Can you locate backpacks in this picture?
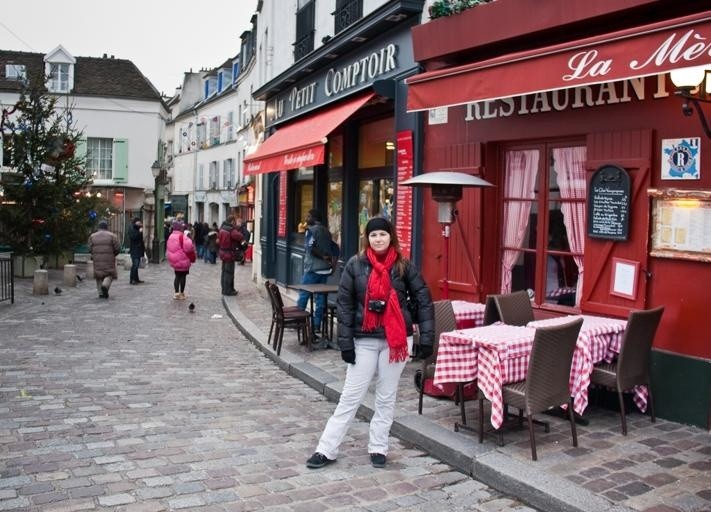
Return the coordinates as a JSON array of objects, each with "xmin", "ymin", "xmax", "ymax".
[
  {"xmin": 185, "ymin": 249, "xmax": 197, "ymax": 263},
  {"xmin": 235, "ymin": 241, "xmax": 248, "ymax": 263}
]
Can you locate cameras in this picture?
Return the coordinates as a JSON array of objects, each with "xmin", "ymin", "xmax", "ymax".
[{"xmin": 368, "ymin": 299, "xmax": 386, "ymax": 316}]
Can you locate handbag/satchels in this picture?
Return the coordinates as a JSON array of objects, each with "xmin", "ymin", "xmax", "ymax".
[
  {"xmin": 407, "ymin": 298, "xmax": 420, "ymax": 324},
  {"xmin": 139, "ymin": 257, "xmax": 146, "ymax": 269}
]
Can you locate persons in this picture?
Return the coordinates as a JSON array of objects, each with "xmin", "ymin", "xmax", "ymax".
[
  {"xmin": 165, "ymin": 215, "xmax": 249, "ymax": 300},
  {"xmin": 306, "ymin": 218, "xmax": 435, "ymax": 469},
  {"xmin": 128, "ymin": 218, "xmax": 144, "ymax": 282},
  {"xmin": 297, "ymin": 210, "xmax": 339, "ymax": 333},
  {"xmin": 88, "ymin": 221, "xmax": 121, "ymax": 298}
]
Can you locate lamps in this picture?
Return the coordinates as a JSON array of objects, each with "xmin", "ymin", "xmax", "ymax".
[
  {"xmin": 670, "ymin": 65, "xmax": 711, "ymax": 117},
  {"xmin": 398, "ymin": 172, "xmax": 496, "ymax": 398}
]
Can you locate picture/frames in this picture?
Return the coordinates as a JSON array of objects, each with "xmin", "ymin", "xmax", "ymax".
[
  {"xmin": 609, "ymin": 257, "xmax": 640, "ymax": 300},
  {"xmin": 647, "ymin": 189, "xmax": 711, "ymax": 262}
]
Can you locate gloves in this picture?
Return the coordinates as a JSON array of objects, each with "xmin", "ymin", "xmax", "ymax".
[
  {"xmin": 416, "ymin": 346, "xmax": 434, "ymax": 359},
  {"xmin": 341, "ymin": 349, "xmax": 356, "ymax": 365}
]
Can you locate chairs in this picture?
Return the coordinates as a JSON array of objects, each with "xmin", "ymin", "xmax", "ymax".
[
  {"xmin": 418, "ymin": 300, "xmax": 467, "ymax": 423},
  {"xmin": 479, "ymin": 318, "xmax": 584, "ymax": 460},
  {"xmin": 589, "ymin": 306, "xmax": 665, "ymax": 435},
  {"xmin": 483, "ymin": 294, "xmax": 500, "ymax": 326},
  {"xmin": 330, "ymin": 313, "xmax": 339, "ymax": 345},
  {"xmin": 268, "ymin": 284, "xmax": 313, "ymax": 355},
  {"xmin": 266, "ymin": 281, "xmax": 304, "ymax": 344},
  {"xmin": 494, "ymin": 290, "xmax": 535, "ymax": 327},
  {"xmin": 322, "ymin": 303, "xmax": 338, "ymax": 338}
]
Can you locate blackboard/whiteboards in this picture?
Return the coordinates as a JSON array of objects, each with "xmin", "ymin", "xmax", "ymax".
[{"xmin": 587, "ymin": 164, "xmax": 632, "ymax": 243}]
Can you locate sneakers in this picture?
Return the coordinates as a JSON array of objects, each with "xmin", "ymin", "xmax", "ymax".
[
  {"xmin": 99, "ymin": 295, "xmax": 103, "ymax": 299},
  {"xmin": 370, "ymin": 453, "xmax": 386, "ymax": 468},
  {"xmin": 306, "ymin": 453, "xmax": 337, "ymax": 469},
  {"xmin": 179, "ymin": 293, "xmax": 186, "ymax": 300},
  {"xmin": 101, "ymin": 285, "xmax": 109, "ymax": 298},
  {"xmin": 173, "ymin": 293, "xmax": 179, "ymax": 300}
]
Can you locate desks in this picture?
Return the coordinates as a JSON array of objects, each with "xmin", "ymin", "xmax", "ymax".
[{"xmin": 285, "ymin": 283, "xmax": 339, "ymax": 348}]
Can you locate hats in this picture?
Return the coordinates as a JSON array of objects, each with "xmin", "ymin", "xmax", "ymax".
[
  {"xmin": 98, "ymin": 221, "xmax": 107, "ymax": 229},
  {"xmin": 172, "ymin": 220, "xmax": 182, "ymax": 230}
]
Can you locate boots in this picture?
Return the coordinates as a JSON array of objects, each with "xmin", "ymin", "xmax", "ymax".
[{"xmin": 129, "ymin": 276, "xmax": 144, "ymax": 285}]
